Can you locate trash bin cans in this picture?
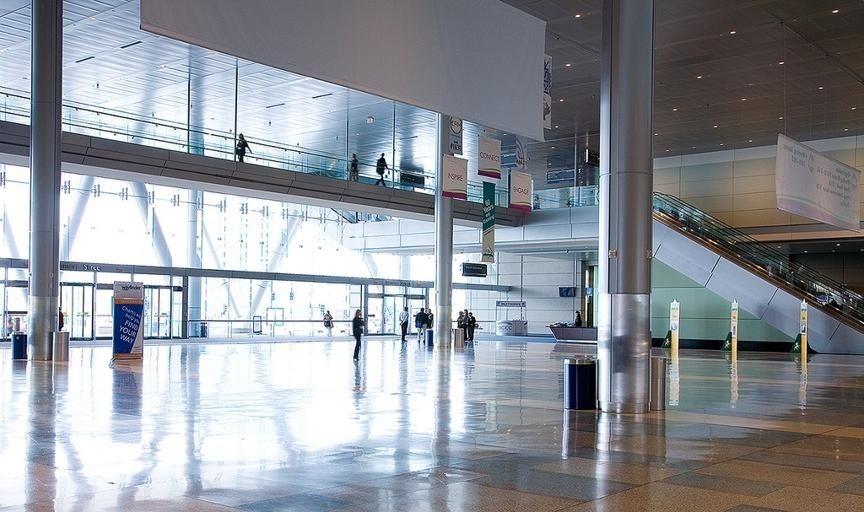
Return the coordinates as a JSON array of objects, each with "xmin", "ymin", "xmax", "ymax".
[
  {"xmin": 452, "ymin": 328, "xmax": 464, "ymax": 350},
  {"xmin": 425, "ymin": 329, "xmax": 433, "ymax": 347},
  {"xmin": 52, "ymin": 331, "xmax": 68, "ymax": 362},
  {"xmin": 563, "ymin": 358, "xmax": 597, "ymax": 411},
  {"xmin": 649, "ymin": 357, "xmax": 666, "ymax": 411},
  {"xmin": 201, "ymin": 323, "xmax": 207, "ymax": 337},
  {"xmin": 10, "ymin": 331, "xmax": 28, "ymax": 359}
]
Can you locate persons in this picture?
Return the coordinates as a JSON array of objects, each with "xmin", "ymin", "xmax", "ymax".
[
  {"xmin": 350, "ymin": 152, "xmax": 360, "ymax": 182},
  {"xmin": 457, "ymin": 311, "xmax": 465, "ymax": 328},
  {"xmin": 236, "ymin": 132, "xmax": 253, "ymax": 163},
  {"xmin": 459, "ymin": 308, "xmax": 471, "ymax": 343},
  {"xmin": 351, "ymin": 308, "xmax": 365, "ymax": 361},
  {"xmin": 574, "ymin": 310, "xmax": 582, "ymax": 328},
  {"xmin": 322, "ymin": 310, "xmax": 335, "ymax": 337},
  {"xmin": 373, "ymin": 152, "xmax": 390, "ymax": 188},
  {"xmin": 426, "ymin": 309, "xmax": 433, "ymax": 329},
  {"xmin": 399, "ymin": 306, "xmax": 409, "ymax": 342},
  {"xmin": 468, "ymin": 311, "xmax": 476, "ymax": 341},
  {"xmin": 414, "ymin": 307, "xmax": 430, "ymax": 344}
]
